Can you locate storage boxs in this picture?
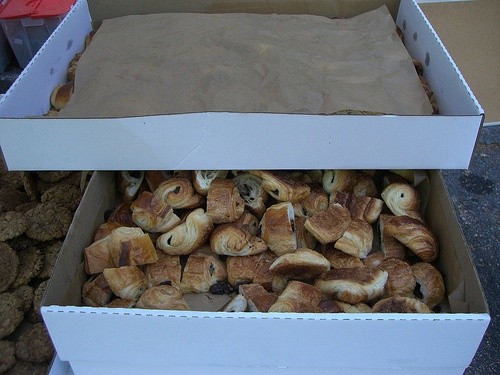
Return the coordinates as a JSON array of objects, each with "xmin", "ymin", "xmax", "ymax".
[
  {"xmin": 1, "ymin": 1, "xmax": 81, "ymax": 75},
  {"xmin": 0, "ymin": 0, "xmax": 491, "ymax": 374}
]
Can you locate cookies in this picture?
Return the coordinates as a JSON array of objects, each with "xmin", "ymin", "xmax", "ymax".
[{"xmin": 0, "ymin": 146, "xmax": 95, "ymax": 375}]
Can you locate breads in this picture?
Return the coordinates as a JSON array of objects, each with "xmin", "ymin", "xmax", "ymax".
[
  {"xmin": 412, "ymin": 59, "xmax": 439, "ymax": 115},
  {"xmin": 81, "ymin": 170, "xmax": 445, "ymax": 313},
  {"xmin": 42, "ymin": 31, "xmax": 97, "ymax": 118},
  {"xmin": 396, "ymin": 25, "xmax": 404, "ymax": 44}
]
